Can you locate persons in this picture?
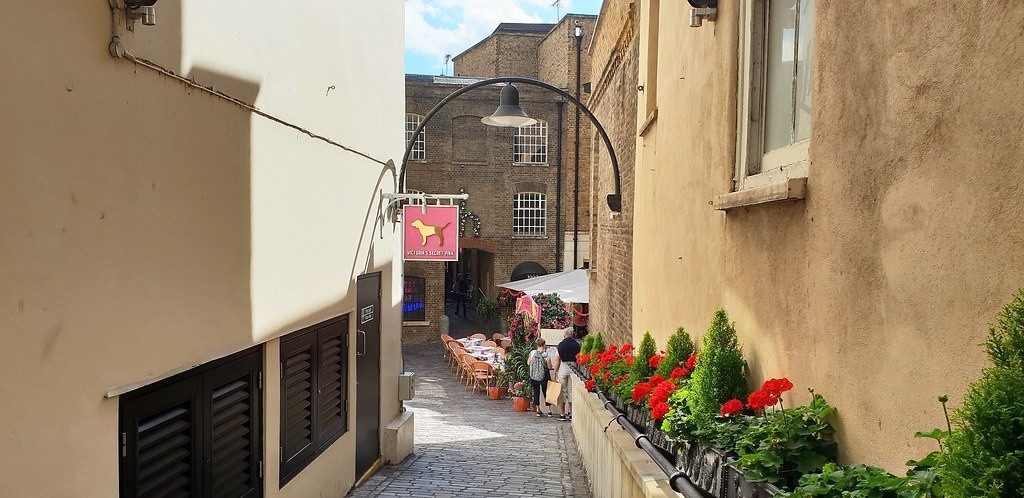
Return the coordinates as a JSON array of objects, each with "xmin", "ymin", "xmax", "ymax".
[
  {"xmin": 529, "ymin": 303, "xmax": 589, "ymax": 421},
  {"xmin": 453, "ymin": 273, "xmax": 468, "ymax": 319}
]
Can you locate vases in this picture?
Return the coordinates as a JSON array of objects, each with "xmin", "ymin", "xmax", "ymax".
[
  {"xmin": 513, "ymin": 397, "xmax": 530, "ymax": 411},
  {"xmin": 489, "ymin": 387, "xmax": 503, "ymax": 399},
  {"xmin": 541, "ymin": 327, "xmax": 573, "ymax": 346},
  {"xmin": 577, "ymin": 364, "xmax": 802, "ymax": 498}
]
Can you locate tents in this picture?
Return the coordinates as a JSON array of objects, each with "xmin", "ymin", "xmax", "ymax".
[{"xmin": 496, "ymin": 269, "xmax": 590, "ymax": 303}]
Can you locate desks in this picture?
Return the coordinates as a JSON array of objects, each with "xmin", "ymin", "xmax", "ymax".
[{"xmin": 457, "ymin": 337, "xmax": 511, "ymax": 372}]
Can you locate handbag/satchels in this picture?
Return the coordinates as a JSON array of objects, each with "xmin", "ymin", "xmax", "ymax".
[
  {"xmin": 544, "ymin": 366, "xmax": 550, "ymax": 379},
  {"xmin": 546, "ymin": 380, "xmax": 564, "ymax": 407}
]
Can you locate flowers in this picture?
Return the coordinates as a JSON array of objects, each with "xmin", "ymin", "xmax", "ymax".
[
  {"xmin": 576, "ymin": 307, "xmax": 837, "ymax": 490},
  {"xmin": 532, "ymin": 292, "xmax": 573, "ymax": 330},
  {"xmin": 488, "ymin": 310, "xmax": 541, "ymax": 402}
]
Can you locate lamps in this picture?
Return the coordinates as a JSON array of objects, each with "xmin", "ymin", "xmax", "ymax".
[{"xmin": 481, "ymin": 81, "xmax": 537, "ymax": 127}]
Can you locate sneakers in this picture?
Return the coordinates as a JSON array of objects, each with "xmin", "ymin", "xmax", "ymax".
[
  {"xmin": 555, "ymin": 413, "xmax": 566, "ymax": 421},
  {"xmin": 547, "ymin": 412, "xmax": 552, "ymax": 417},
  {"xmin": 570, "ymin": 416, "xmax": 572, "ymax": 420},
  {"xmin": 537, "ymin": 411, "xmax": 544, "ymax": 417}
]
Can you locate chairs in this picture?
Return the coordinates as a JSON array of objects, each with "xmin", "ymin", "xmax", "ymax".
[
  {"xmin": 471, "ymin": 333, "xmax": 512, "ymax": 360},
  {"xmin": 441, "ymin": 334, "xmax": 495, "ymax": 396}
]
